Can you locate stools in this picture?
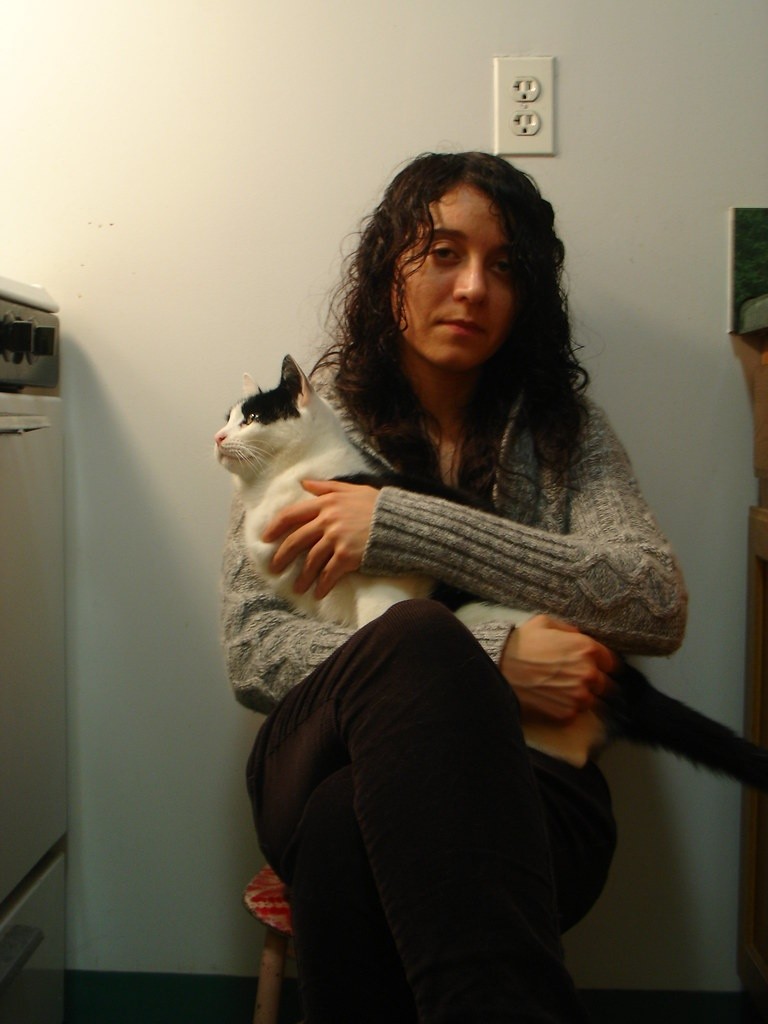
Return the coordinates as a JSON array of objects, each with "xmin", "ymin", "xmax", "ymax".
[{"xmin": 241, "ymin": 862, "xmax": 293, "ymax": 1024}]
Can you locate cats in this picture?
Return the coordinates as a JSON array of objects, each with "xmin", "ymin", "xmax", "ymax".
[{"xmin": 213, "ymin": 348, "xmax": 768, "ymax": 794}]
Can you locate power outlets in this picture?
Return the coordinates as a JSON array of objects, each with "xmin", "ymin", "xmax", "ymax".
[{"xmin": 492, "ymin": 55, "xmax": 558, "ymax": 156}]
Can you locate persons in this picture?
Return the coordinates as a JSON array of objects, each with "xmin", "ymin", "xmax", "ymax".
[{"xmin": 219, "ymin": 151, "xmax": 688, "ymax": 1024}]
